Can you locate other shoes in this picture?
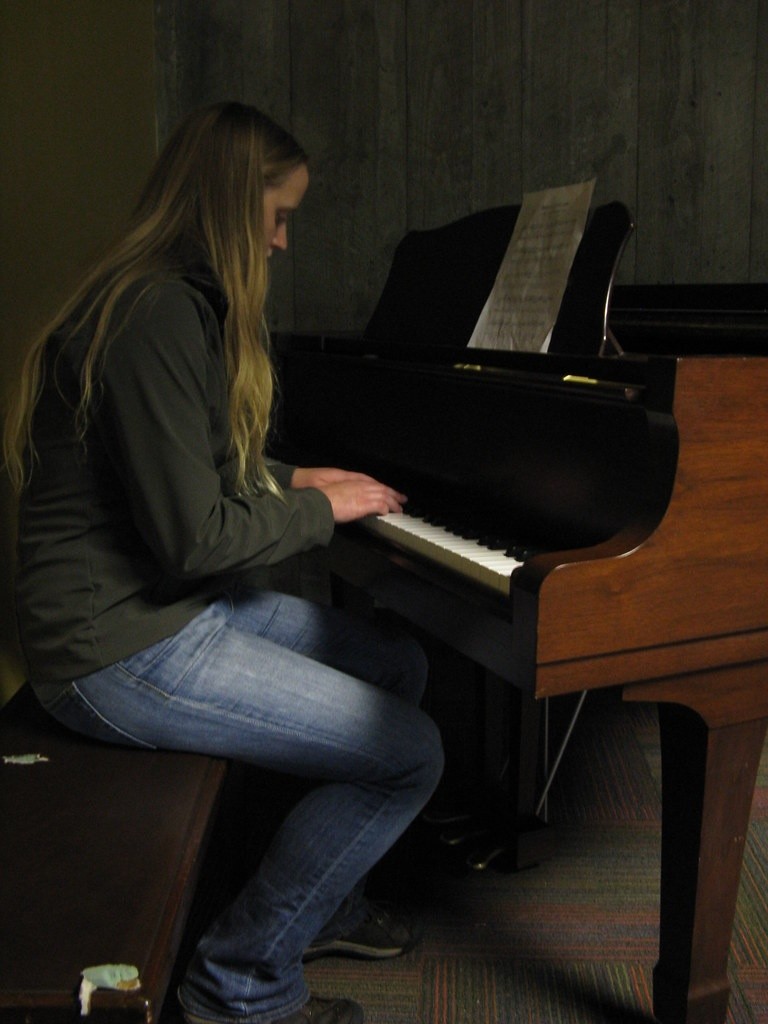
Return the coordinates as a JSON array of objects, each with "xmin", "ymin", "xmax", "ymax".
[
  {"xmin": 302, "ymin": 915, "xmax": 404, "ymax": 958},
  {"xmin": 268, "ymin": 993, "xmax": 367, "ymax": 1024}
]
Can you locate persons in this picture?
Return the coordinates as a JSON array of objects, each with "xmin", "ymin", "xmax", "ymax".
[{"xmin": 1, "ymin": 102, "xmax": 452, "ymax": 1024}]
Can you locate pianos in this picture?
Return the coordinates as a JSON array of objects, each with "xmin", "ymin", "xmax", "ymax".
[{"xmin": 267, "ymin": 278, "xmax": 768, "ymax": 1024}]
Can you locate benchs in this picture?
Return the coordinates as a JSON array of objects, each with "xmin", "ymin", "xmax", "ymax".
[{"xmin": 1, "ymin": 676, "xmax": 238, "ymax": 1024}]
